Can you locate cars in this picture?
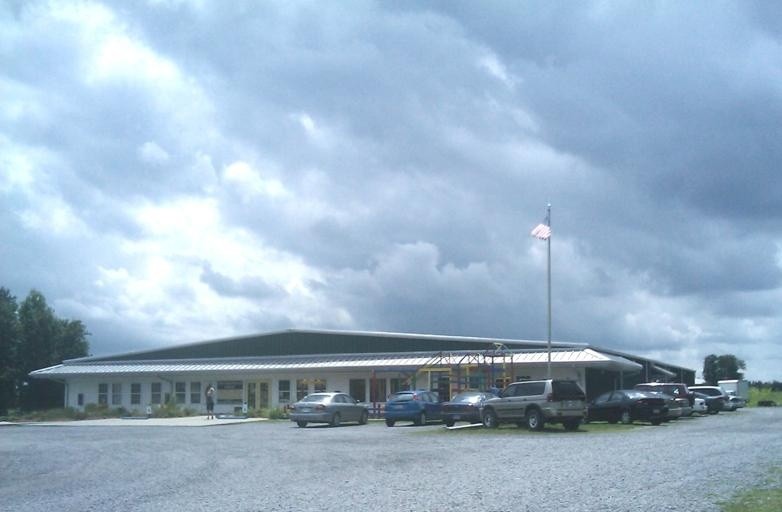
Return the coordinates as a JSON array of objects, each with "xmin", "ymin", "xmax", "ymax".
[
  {"xmin": 439, "ymin": 392, "xmax": 496, "ymax": 427},
  {"xmin": 689, "ymin": 380, "xmax": 747, "ymax": 415},
  {"xmin": 583, "ymin": 390, "xmax": 669, "ymax": 425},
  {"xmin": 648, "ymin": 390, "xmax": 691, "ymax": 420},
  {"xmin": 289, "ymin": 392, "xmax": 369, "ymax": 427},
  {"xmin": 383, "ymin": 390, "xmax": 446, "ymax": 426}
]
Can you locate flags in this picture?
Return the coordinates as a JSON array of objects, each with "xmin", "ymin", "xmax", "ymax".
[{"xmin": 530, "ymin": 211, "xmax": 552, "ymax": 241}]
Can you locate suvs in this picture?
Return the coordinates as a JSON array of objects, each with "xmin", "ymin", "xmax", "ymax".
[
  {"xmin": 632, "ymin": 382, "xmax": 696, "ymax": 416},
  {"xmin": 479, "ymin": 379, "xmax": 588, "ymax": 431}
]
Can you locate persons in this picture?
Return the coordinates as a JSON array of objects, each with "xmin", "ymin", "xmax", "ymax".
[{"xmin": 206, "ymin": 387, "xmax": 215, "ymax": 420}]
